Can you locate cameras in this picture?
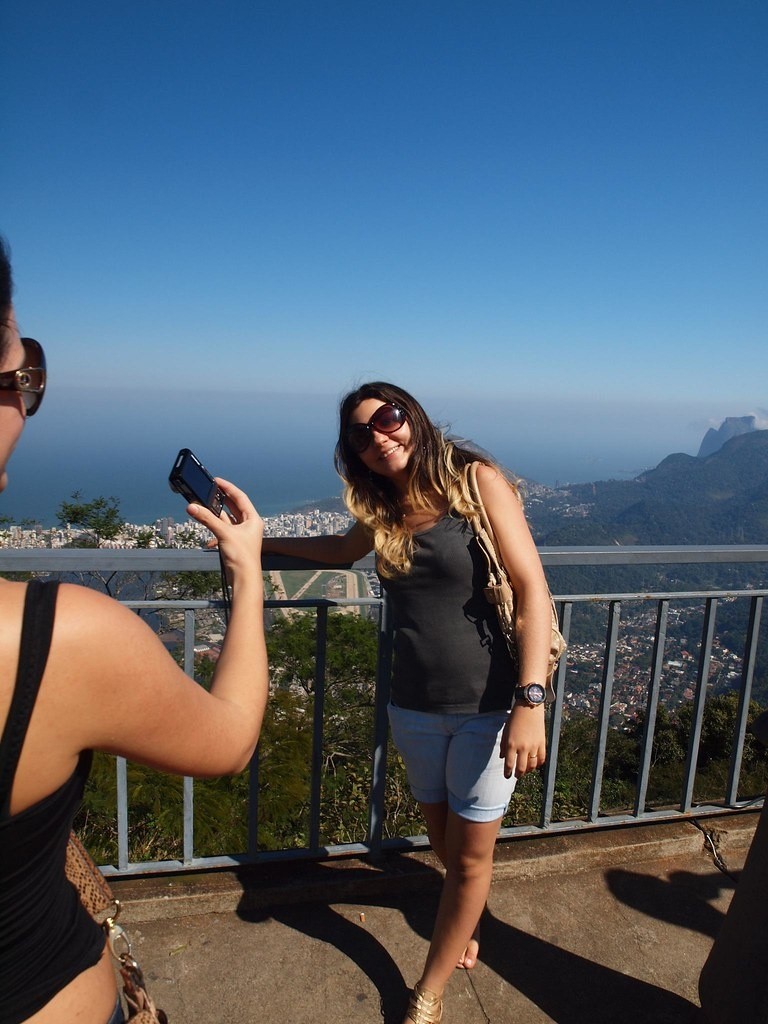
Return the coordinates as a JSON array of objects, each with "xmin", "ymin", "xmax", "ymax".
[{"xmin": 168, "ymin": 448, "xmax": 228, "ymax": 518}]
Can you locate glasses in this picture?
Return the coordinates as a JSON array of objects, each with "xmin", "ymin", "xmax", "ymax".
[
  {"xmin": 0, "ymin": 338, "xmax": 47, "ymax": 416},
  {"xmin": 347, "ymin": 403, "xmax": 406, "ymax": 452}
]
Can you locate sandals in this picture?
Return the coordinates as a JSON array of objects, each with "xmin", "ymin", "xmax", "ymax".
[{"xmin": 407, "ymin": 981, "xmax": 443, "ymax": 1024}]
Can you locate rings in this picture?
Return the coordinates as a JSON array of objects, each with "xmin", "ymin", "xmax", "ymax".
[{"xmin": 529, "ymin": 755, "xmax": 537, "ymax": 758}]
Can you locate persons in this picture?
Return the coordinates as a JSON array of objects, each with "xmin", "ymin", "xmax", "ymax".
[
  {"xmin": 204, "ymin": 381, "xmax": 554, "ymax": 1024},
  {"xmin": 1, "ymin": 240, "xmax": 269, "ymax": 1023}
]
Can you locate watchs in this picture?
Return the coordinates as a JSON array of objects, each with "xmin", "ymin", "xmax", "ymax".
[{"xmin": 515, "ymin": 682, "xmax": 547, "ymax": 708}]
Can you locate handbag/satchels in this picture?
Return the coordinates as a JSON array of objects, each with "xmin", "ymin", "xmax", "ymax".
[
  {"xmin": 459, "ymin": 460, "xmax": 567, "ymax": 675},
  {"xmin": 119, "ymin": 970, "xmax": 160, "ymax": 1024}
]
